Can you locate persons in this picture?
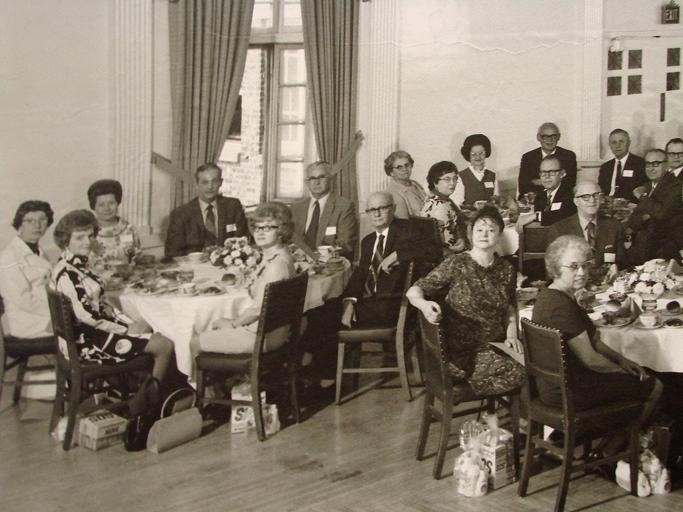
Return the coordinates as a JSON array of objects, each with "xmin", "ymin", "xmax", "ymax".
[
  {"xmin": 547, "ymin": 179, "xmax": 626, "ymax": 271},
  {"xmin": 628, "ymin": 148, "xmax": 683, "ymax": 271},
  {"xmin": 518, "ymin": 123, "xmax": 576, "ymax": 201},
  {"xmin": 534, "ymin": 154, "xmax": 577, "ymax": 225},
  {"xmin": 165, "ymin": 162, "xmax": 255, "ymax": 264},
  {"xmin": 47, "ymin": 206, "xmax": 173, "ymax": 417},
  {"xmin": 406, "ymin": 206, "xmax": 525, "ymax": 397},
  {"xmin": 284, "ymin": 160, "xmax": 359, "ymax": 266},
  {"xmin": 449, "ymin": 134, "xmax": 500, "ymax": 212},
  {"xmin": 87, "ymin": 179, "xmax": 154, "ymax": 283},
  {"xmin": 187, "ymin": 202, "xmax": 296, "ymax": 425},
  {"xmin": 385, "ymin": 151, "xmax": 428, "ymax": 218},
  {"xmin": 597, "ymin": 129, "xmax": 648, "ymax": 200},
  {"xmin": 665, "ymin": 138, "xmax": 683, "ymax": 180},
  {"xmin": 0, "ymin": 200, "xmax": 55, "ymax": 342},
  {"xmin": 421, "ymin": 161, "xmax": 470, "ymax": 255},
  {"xmin": 532, "ymin": 233, "xmax": 663, "ymax": 474},
  {"xmin": 302, "ymin": 192, "xmax": 431, "ymax": 404}
]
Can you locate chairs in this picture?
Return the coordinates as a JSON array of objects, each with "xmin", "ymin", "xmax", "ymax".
[
  {"xmin": 414, "ymin": 308, "xmax": 524, "ymax": 483},
  {"xmin": 195, "ymin": 269, "xmax": 308, "ymax": 442},
  {"xmin": 518, "ymin": 315, "xmax": 644, "ymax": 512},
  {"xmin": 335, "ymin": 258, "xmax": 425, "ymax": 405},
  {"xmin": 41, "ymin": 281, "xmax": 153, "ymax": 451},
  {"xmin": 0, "ymin": 295, "xmax": 57, "ymax": 404},
  {"xmin": 517, "ymin": 229, "xmax": 550, "ymax": 286}
]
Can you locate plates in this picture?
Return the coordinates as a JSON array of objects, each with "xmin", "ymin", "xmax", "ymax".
[
  {"xmin": 316, "ymin": 259, "xmax": 327, "ymax": 262},
  {"xmin": 129, "ymin": 251, "xmax": 242, "ymax": 299},
  {"xmin": 586, "ymin": 275, "xmax": 682, "ymax": 331}
]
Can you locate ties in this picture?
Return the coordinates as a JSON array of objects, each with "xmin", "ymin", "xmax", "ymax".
[
  {"xmin": 363, "ymin": 233, "xmax": 385, "ymax": 297},
  {"xmin": 585, "ymin": 221, "xmax": 597, "ymax": 246},
  {"xmin": 203, "ymin": 203, "xmax": 216, "ymax": 246},
  {"xmin": 545, "ymin": 193, "xmax": 552, "ymax": 213}
]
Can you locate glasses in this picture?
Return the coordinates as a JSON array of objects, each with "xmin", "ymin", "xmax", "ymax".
[
  {"xmin": 561, "ymin": 261, "xmax": 593, "ymax": 272},
  {"xmin": 538, "ymin": 134, "xmax": 683, "ymax": 201},
  {"xmin": 253, "ymin": 151, "xmax": 488, "ymax": 233}
]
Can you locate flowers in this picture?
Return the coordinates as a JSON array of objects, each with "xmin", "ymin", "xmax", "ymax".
[
  {"xmin": 611, "ymin": 255, "xmax": 682, "ymax": 296},
  {"xmin": 211, "ymin": 244, "xmax": 261, "ymax": 270}
]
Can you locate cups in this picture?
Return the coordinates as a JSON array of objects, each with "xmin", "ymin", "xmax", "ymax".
[
  {"xmin": 519, "ymin": 287, "xmax": 538, "ymax": 298},
  {"xmin": 475, "ymin": 200, "xmax": 486, "ymax": 210},
  {"xmin": 502, "ymin": 217, "xmax": 510, "ymax": 229},
  {"xmin": 317, "ymin": 245, "xmax": 334, "ymax": 257}
]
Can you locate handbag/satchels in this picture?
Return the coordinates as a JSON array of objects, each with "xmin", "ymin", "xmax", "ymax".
[
  {"xmin": 20, "ymin": 355, "xmax": 68, "ymax": 401},
  {"xmin": 147, "ymin": 388, "xmax": 203, "ymax": 453},
  {"xmin": 125, "ymin": 376, "xmax": 171, "ymax": 451}
]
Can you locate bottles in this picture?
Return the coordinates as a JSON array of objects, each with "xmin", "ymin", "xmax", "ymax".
[
  {"xmin": 456, "ymin": 462, "xmax": 489, "ymax": 497},
  {"xmin": 639, "ymin": 454, "xmax": 671, "ymax": 496},
  {"xmin": 267, "ymin": 403, "xmax": 279, "ymax": 433}
]
